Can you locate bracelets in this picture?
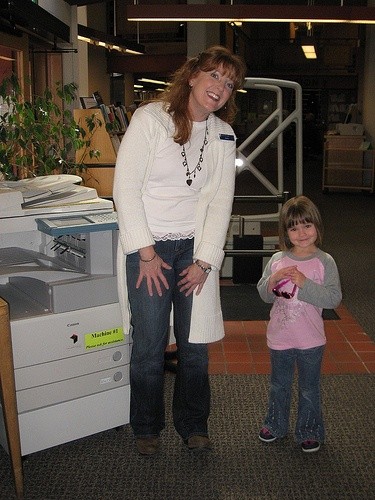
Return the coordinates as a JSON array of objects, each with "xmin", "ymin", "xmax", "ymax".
[{"xmin": 140, "ymin": 253, "xmax": 157, "ymax": 262}]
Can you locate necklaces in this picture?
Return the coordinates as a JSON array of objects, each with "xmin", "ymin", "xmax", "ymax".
[{"xmin": 181, "ymin": 117, "xmax": 209, "ymax": 187}]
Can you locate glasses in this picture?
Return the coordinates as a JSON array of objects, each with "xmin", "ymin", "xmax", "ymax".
[{"xmin": 272, "ymin": 278, "xmax": 297, "ymax": 299}]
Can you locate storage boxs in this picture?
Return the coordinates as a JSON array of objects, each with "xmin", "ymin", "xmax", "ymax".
[{"xmin": 325, "ymin": 124, "xmax": 375, "ymax": 193}]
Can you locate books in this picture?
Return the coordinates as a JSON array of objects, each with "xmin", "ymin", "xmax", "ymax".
[{"xmin": 86, "ymin": 104, "xmax": 130, "ymax": 132}]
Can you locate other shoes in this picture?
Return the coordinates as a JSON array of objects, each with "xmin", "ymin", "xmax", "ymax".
[
  {"xmin": 136, "ymin": 437, "xmax": 160, "ymax": 455},
  {"xmin": 188, "ymin": 436, "xmax": 212, "ymax": 451},
  {"xmin": 301, "ymin": 440, "xmax": 320, "ymax": 452},
  {"xmin": 259, "ymin": 427, "xmax": 276, "ymax": 442}
]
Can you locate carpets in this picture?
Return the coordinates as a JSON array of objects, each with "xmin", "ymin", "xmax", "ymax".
[
  {"xmin": 220, "ymin": 285, "xmax": 342, "ymax": 324},
  {"xmin": 0, "ymin": 374, "xmax": 375, "ymax": 500}
]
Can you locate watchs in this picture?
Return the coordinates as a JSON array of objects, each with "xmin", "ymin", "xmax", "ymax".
[{"xmin": 195, "ymin": 260, "xmax": 212, "ymax": 273}]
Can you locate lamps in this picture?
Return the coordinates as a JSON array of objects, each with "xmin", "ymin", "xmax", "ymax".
[
  {"xmin": 78, "ymin": 0, "xmax": 145, "ymax": 55},
  {"xmin": 127, "ymin": 0, "xmax": 375, "ymax": 24}
]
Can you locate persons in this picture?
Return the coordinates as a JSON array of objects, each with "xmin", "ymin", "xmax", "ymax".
[
  {"xmin": 111, "ymin": 46, "xmax": 246, "ymax": 459},
  {"xmin": 257, "ymin": 194, "xmax": 341, "ymax": 453}
]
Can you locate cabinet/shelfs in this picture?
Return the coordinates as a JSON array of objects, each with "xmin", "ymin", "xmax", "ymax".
[
  {"xmin": 321, "ymin": 142, "xmax": 375, "ymax": 196},
  {"xmin": 1, "ymin": 198, "xmax": 133, "ymax": 457}
]
